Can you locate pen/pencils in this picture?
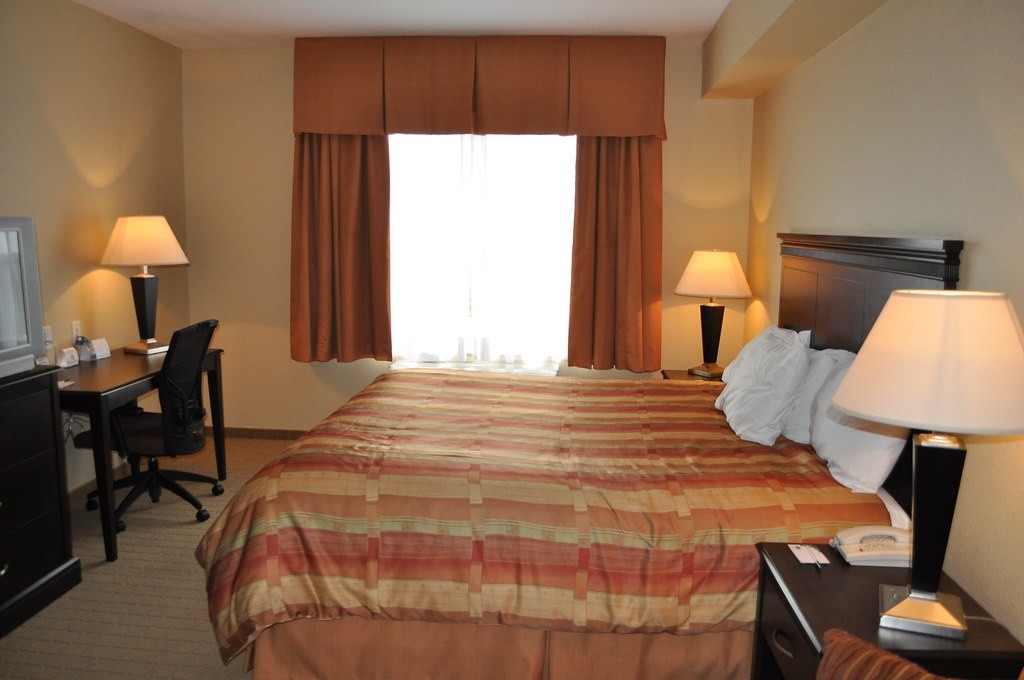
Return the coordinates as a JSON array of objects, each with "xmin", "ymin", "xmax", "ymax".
[{"xmin": 804, "ymin": 543, "xmax": 822, "ymax": 568}]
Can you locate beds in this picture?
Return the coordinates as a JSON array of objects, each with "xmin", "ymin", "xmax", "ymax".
[{"xmin": 194, "ymin": 233, "xmax": 967, "ymax": 680}]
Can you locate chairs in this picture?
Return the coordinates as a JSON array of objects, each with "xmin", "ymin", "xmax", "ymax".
[{"xmin": 73, "ymin": 319, "xmax": 225, "ymax": 530}]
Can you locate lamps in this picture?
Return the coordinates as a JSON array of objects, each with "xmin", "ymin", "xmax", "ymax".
[
  {"xmin": 832, "ymin": 289, "xmax": 1024, "ymax": 638},
  {"xmin": 100, "ymin": 215, "xmax": 190, "ymax": 356},
  {"xmin": 674, "ymin": 250, "xmax": 753, "ymax": 378}
]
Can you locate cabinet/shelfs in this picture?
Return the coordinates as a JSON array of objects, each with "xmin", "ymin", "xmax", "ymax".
[{"xmin": 1, "ymin": 365, "xmax": 83, "ymax": 636}]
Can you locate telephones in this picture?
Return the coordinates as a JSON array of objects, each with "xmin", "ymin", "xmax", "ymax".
[{"xmin": 835, "ymin": 524, "xmax": 913, "ymax": 567}]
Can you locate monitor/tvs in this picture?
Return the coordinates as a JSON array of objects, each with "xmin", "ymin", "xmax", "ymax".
[{"xmin": 0, "ymin": 216, "xmax": 46, "ymax": 378}]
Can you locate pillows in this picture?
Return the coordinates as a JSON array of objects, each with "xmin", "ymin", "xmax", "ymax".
[
  {"xmin": 781, "ymin": 349, "xmax": 844, "ymax": 446},
  {"xmin": 809, "ymin": 348, "xmax": 912, "ymax": 493},
  {"xmin": 721, "ymin": 324, "xmax": 811, "ymax": 383},
  {"xmin": 715, "ymin": 333, "xmax": 811, "ymax": 446}
]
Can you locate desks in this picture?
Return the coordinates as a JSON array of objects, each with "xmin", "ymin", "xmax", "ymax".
[{"xmin": 57, "ymin": 345, "xmax": 228, "ymax": 562}]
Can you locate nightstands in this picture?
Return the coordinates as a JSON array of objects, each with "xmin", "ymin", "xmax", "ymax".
[
  {"xmin": 749, "ymin": 543, "xmax": 1024, "ymax": 680},
  {"xmin": 661, "ymin": 370, "xmax": 723, "ymax": 383}
]
